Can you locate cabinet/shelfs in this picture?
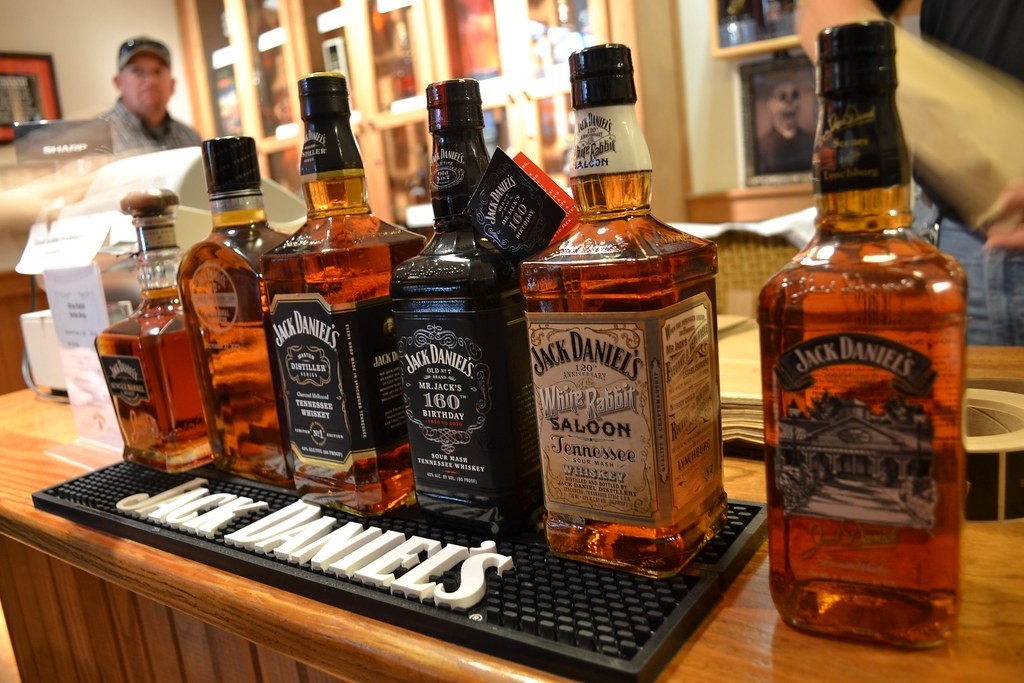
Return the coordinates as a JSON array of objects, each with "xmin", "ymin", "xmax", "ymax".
[{"xmin": 174, "ymin": 0, "xmax": 696, "ymax": 225}]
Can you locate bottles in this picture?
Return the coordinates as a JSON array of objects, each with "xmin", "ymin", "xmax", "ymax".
[
  {"xmin": 94, "ymin": 189, "xmax": 214, "ymax": 473},
  {"xmin": 757, "ymin": 20, "xmax": 969, "ymax": 651},
  {"xmin": 329, "ymin": 46, "xmax": 340, "ymax": 73},
  {"xmin": 176, "ymin": 136, "xmax": 292, "ymax": 488},
  {"xmin": 388, "ymin": 79, "xmax": 548, "ymax": 536},
  {"xmin": 404, "ymin": 123, "xmax": 433, "ymax": 205},
  {"xmin": 519, "ymin": 42, "xmax": 729, "ymax": 579},
  {"xmin": 256, "ymin": 72, "xmax": 427, "ymax": 517},
  {"xmin": 389, "ymin": 7, "xmax": 417, "ymax": 98}
]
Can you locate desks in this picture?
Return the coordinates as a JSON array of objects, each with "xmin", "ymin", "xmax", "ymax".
[{"xmin": 2, "ymin": 368, "xmax": 1024, "ymax": 682}]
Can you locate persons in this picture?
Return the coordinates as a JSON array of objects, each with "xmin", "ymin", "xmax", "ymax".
[
  {"xmin": 795, "ymin": 0, "xmax": 1024, "ymax": 347},
  {"xmin": 758, "ymin": 80, "xmax": 813, "ymax": 175},
  {"xmin": 98, "ymin": 37, "xmax": 203, "ymax": 156}
]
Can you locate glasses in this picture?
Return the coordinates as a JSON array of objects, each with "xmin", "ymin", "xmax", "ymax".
[{"xmin": 119, "ymin": 40, "xmax": 164, "ymax": 63}]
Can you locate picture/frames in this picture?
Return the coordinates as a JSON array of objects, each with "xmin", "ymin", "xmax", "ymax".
[
  {"xmin": 710, "ymin": 0, "xmax": 802, "ymax": 57},
  {"xmin": 732, "ymin": 57, "xmax": 814, "ymax": 185},
  {"xmin": 0, "ymin": 50, "xmax": 62, "ymax": 143}
]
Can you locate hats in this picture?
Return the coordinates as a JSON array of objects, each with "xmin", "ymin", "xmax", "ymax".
[{"xmin": 118, "ymin": 36, "xmax": 170, "ymax": 72}]
